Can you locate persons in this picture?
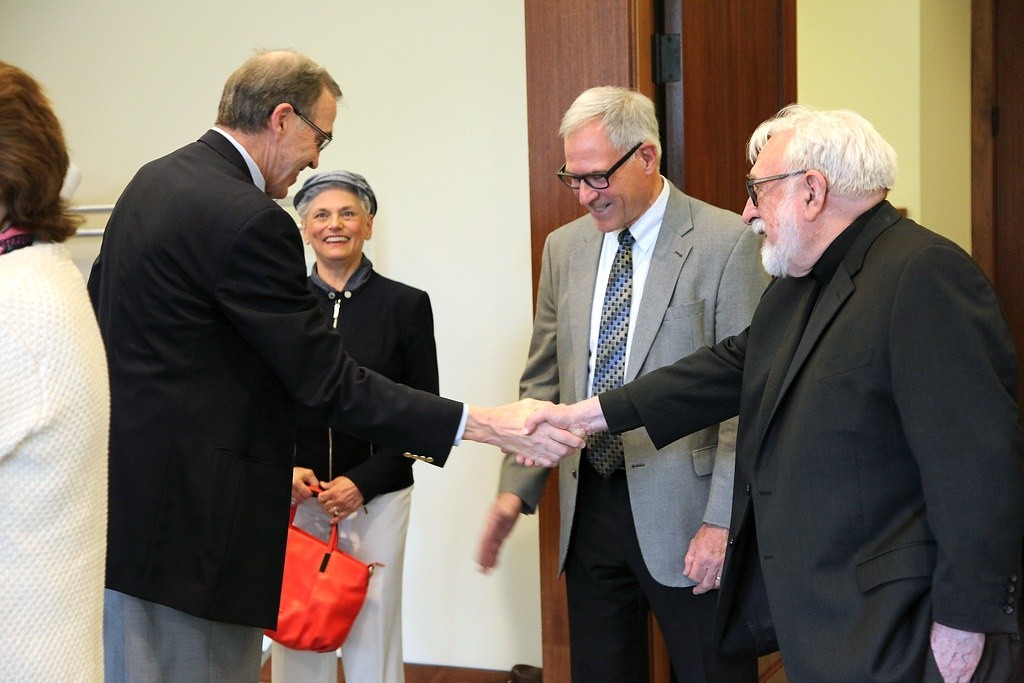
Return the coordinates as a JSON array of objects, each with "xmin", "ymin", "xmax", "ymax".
[
  {"xmin": 476, "ymin": 85, "xmax": 782, "ymax": 683},
  {"xmin": 85, "ymin": 48, "xmax": 589, "ymax": 683},
  {"xmin": 269, "ymin": 168, "xmax": 441, "ymax": 683},
  {"xmin": 0, "ymin": 57, "xmax": 113, "ymax": 681},
  {"xmin": 499, "ymin": 104, "xmax": 1023, "ymax": 683}
]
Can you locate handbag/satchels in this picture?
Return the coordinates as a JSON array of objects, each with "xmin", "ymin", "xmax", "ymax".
[{"xmin": 263, "ymin": 486, "xmax": 385, "ymax": 653}]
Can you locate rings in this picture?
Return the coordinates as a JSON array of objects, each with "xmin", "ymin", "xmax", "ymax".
[
  {"xmin": 335, "ymin": 508, "xmax": 338, "ymax": 517},
  {"xmin": 715, "ymin": 577, "xmax": 721, "ymax": 580}
]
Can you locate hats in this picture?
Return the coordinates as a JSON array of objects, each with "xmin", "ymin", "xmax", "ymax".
[{"xmin": 293, "ymin": 170, "xmax": 377, "ymax": 218}]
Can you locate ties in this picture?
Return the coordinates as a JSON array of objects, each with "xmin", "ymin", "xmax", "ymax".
[{"xmin": 586, "ymin": 227, "xmax": 636, "ymax": 482}]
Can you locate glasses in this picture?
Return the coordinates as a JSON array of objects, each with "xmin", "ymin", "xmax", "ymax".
[
  {"xmin": 556, "ymin": 142, "xmax": 642, "ymax": 189},
  {"xmin": 270, "ymin": 103, "xmax": 333, "ymax": 153},
  {"xmin": 746, "ymin": 169, "xmax": 829, "ymax": 206}
]
